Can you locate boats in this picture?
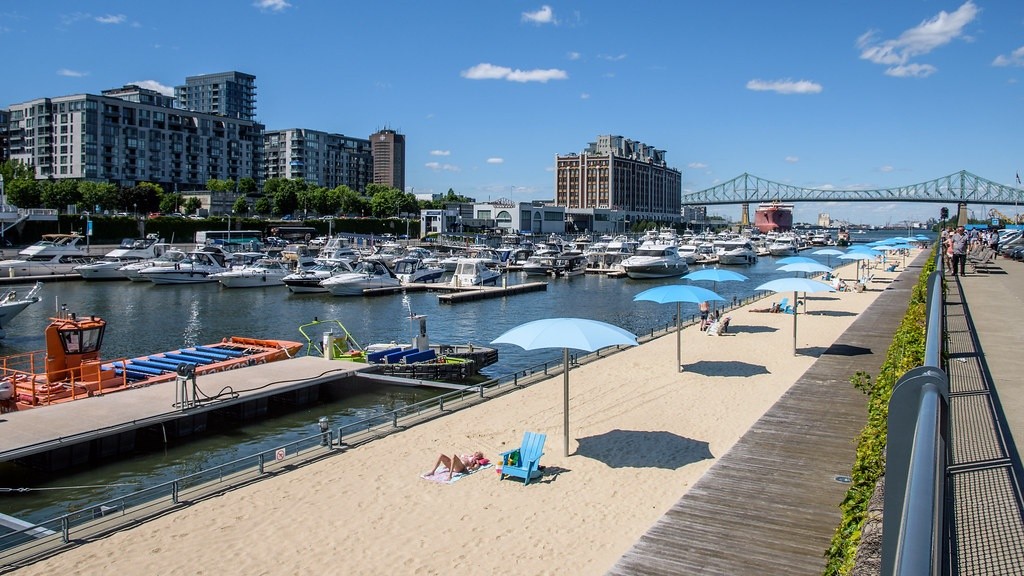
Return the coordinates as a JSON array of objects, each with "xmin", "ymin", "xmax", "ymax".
[
  {"xmin": 200, "ymin": 226, "xmax": 851, "ymax": 279},
  {"xmin": 117, "ymin": 244, "xmax": 188, "ymax": 280},
  {"xmin": 73, "ymin": 235, "xmax": 163, "ymax": 281},
  {"xmin": 450, "ymin": 259, "xmax": 500, "ymax": 288},
  {"xmin": 139, "ymin": 244, "xmax": 238, "ymax": 282},
  {"xmin": 755, "ymin": 202, "xmax": 795, "ymax": 233},
  {"xmin": 226, "ymin": 251, "xmax": 265, "ymax": 271},
  {"xmin": 390, "ymin": 259, "xmax": 447, "ymax": 285},
  {"xmin": 207, "ymin": 255, "xmax": 290, "ymax": 289},
  {"xmin": 282, "ymin": 257, "xmax": 354, "ymax": 293},
  {"xmin": 0, "ymin": 282, "xmax": 302, "ymax": 416},
  {"xmin": 301, "ymin": 314, "xmax": 498, "ymax": 381},
  {"xmin": 0, "ymin": 232, "xmax": 98, "ymax": 282},
  {"xmin": 319, "ymin": 259, "xmax": 404, "ymax": 295}
]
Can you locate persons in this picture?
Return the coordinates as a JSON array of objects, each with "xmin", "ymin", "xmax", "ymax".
[
  {"xmin": 941, "ymin": 225, "xmax": 1000, "ymax": 277},
  {"xmin": 699, "ymin": 301, "xmax": 710, "ymax": 331},
  {"xmin": 821, "ymin": 248, "xmax": 910, "ymax": 293},
  {"xmin": 424, "ymin": 451, "xmax": 484, "ymax": 480}
]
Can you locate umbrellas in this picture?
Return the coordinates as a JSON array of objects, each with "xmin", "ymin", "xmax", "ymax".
[
  {"xmin": 680, "ymin": 269, "xmax": 749, "ymax": 319},
  {"xmin": 754, "ymin": 278, "xmax": 836, "ymax": 357},
  {"xmin": 813, "ymin": 233, "xmax": 930, "ymax": 281},
  {"xmin": 776, "ymin": 263, "xmax": 833, "ymax": 314},
  {"xmin": 632, "ymin": 285, "xmax": 725, "ymax": 374},
  {"xmin": 776, "ymin": 257, "xmax": 819, "ymax": 277},
  {"xmin": 489, "ymin": 317, "xmax": 639, "ymax": 457}
]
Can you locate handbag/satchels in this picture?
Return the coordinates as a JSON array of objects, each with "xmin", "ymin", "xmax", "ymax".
[{"xmin": 944, "ymin": 255, "xmax": 949, "ymax": 262}]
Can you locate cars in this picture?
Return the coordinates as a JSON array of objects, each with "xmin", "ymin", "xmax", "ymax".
[{"xmin": 82, "ymin": 212, "xmax": 378, "ymax": 220}]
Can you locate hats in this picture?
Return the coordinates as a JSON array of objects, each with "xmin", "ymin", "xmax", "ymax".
[{"xmin": 950, "ymin": 232, "xmax": 955, "ymax": 235}]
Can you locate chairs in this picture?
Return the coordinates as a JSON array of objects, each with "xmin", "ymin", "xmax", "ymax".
[
  {"xmin": 500, "ymin": 431, "xmax": 546, "ymax": 485},
  {"xmin": 822, "ymin": 248, "xmax": 909, "ymax": 292},
  {"xmin": 967, "ymin": 242, "xmax": 998, "ymax": 274},
  {"xmin": 706, "ymin": 317, "xmax": 730, "ymax": 336},
  {"xmin": 772, "ymin": 297, "xmax": 799, "ymax": 314}
]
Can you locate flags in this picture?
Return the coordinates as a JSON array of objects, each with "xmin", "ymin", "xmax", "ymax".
[{"xmin": 1016, "ymin": 173, "xmax": 1021, "ymax": 184}]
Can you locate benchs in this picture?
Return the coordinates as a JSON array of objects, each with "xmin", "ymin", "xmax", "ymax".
[
  {"xmin": 99, "ymin": 345, "xmax": 242, "ymax": 382},
  {"xmin": 364, "ymin": 347, "xmax": 436, "ymax": 364}
]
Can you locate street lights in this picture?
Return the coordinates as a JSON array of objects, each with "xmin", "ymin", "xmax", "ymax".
[{"xmin": 611, "ymin": 205, "xmax": 625, "ymax": 234}]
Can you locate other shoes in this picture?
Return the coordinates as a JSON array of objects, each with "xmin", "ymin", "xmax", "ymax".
[
  {"xmin": 951, "ymin": 271, "xmax": 957, "ymax": 276},
  {"xmin": 961, "ymin": 274, "xmax": 966, "ymax": 277}
]
